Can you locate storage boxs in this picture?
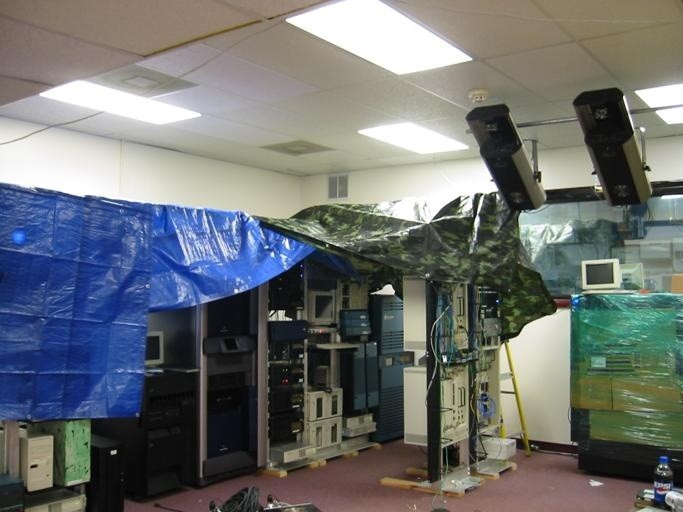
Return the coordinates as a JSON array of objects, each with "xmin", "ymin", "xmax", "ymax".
[{"xmin": 28, "ymin": 419, "xmax": 90, "ymax": 487}]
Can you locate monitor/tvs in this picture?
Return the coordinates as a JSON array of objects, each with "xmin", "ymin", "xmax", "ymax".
[
  {"xmin": 145, "ymin": 330, "xmax": 164, "ymax": 368},
  {"xmin": 308, "ymin": 290, "xmax": 333, "ymax": 326},
  {"xmin": 619, "ymin": 263, "xmax": 644, "ymax": 290},
  {"xmin": 340, "ymin": 309, "xmax": 374, "ymax": 343},
  {"xmin": 580, "ymin": 258, "xmax": 621, "ymax": 290}
]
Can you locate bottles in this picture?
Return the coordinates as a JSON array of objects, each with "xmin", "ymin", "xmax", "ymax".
[{"xmin": 653, "ymin": 455, "xmax": 672, "ymax": 506}]
